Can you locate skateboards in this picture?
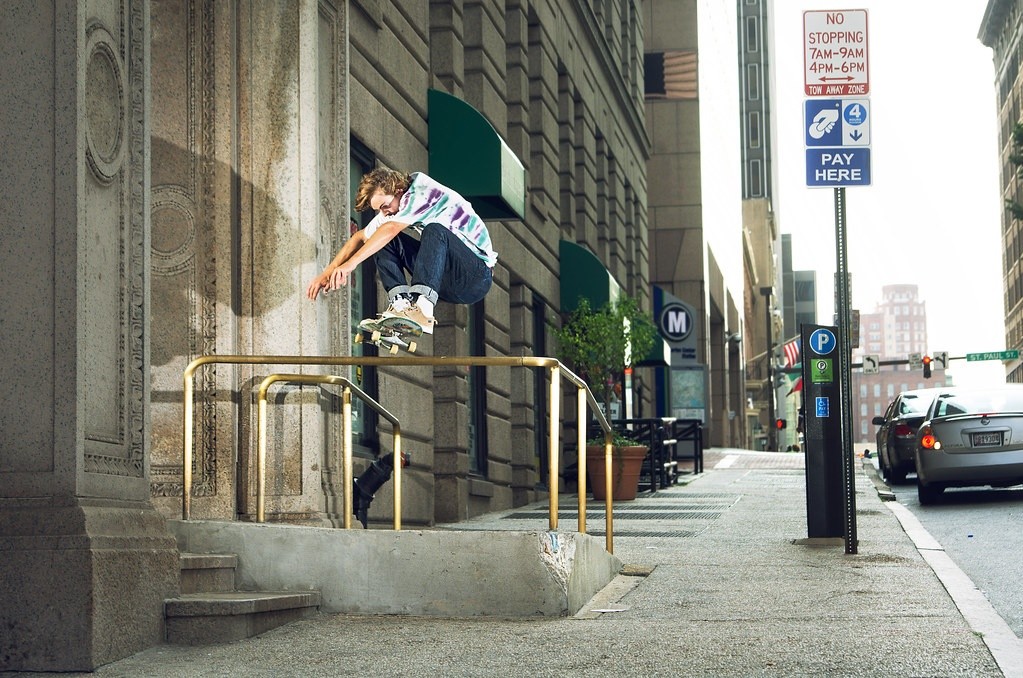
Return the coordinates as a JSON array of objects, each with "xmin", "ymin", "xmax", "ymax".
[{"xmin": 355, "ymin": 316, "xmax": 424, "ymax": 355}]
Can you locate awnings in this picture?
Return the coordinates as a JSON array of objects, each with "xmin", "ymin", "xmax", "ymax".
[
  {"xmin": 428, "ymin": 88, "xmax": 524, "ymax": 221},
  {"xmin": 632, "ymin": 335, "xmax": 671, "ymax": 368},
  {"xmin": 558, "ymin": 239, "xmax": 620, "ymax": 314}
]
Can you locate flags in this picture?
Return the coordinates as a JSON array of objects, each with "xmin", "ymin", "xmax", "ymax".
[
  {"xmin": 786, "ymin": 362, "xmax": 802, "ymax": 397},
  {"xmin": 784, "ymin": 337, "xmax": 801, "ymax": 372}
]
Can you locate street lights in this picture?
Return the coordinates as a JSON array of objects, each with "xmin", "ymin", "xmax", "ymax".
[{"xmin": 759, "ymin": 285, "xmax": 778, "ymax": 452}]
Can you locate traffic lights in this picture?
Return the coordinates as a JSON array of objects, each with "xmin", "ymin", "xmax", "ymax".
[
  {"xmin": 776, "ymin": 418, "xmax": 786, "ymax": 430},
  {"xmin": 923, "ymin": 356, "xmax": 931, "ymax": 378}
]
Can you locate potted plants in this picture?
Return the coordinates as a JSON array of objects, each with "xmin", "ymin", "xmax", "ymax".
[{"xmin": 546, "ymin": 291, "xmax": 659, "ymax": 501}]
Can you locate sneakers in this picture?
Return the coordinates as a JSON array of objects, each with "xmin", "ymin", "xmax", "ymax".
[
  {"xmin": 360, "ymin": 303, "xmax": 411, "ymax": 337},
  {"xmin": 383, "ymin": 303, "xmax": 438, "ymax": 335}
]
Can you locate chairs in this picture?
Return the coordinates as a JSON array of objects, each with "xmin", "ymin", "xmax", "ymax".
[{"xmin": 947, "ymin": 402, "xmax": 966, "ymax": 414}]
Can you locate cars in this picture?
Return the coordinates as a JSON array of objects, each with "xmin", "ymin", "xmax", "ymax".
[
  {"xmin": 872, "ymin": 387, "xmax": 959, "ymax": 485},
  {"xmin": 907, "ymin": 383, "xmax": 1023, "ymax": 506}
]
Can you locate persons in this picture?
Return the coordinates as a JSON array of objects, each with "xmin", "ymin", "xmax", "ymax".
[{"xmin": 307, "ymin": 169, "xmax": 499, "ymax": 335}]
